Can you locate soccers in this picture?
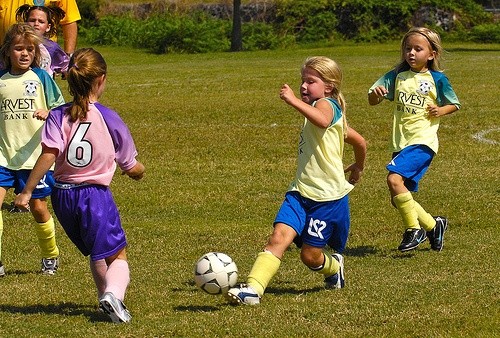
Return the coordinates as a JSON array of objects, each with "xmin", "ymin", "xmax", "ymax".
[{"xmin": 193, "ymin": 251, "xmax": 239, "ymax": 295}]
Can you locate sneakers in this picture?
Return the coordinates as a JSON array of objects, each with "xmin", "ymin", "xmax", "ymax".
[
  {"xmin": 99, "ymin": 291, "xmax": 134, "ymax": 325},
  {"xmin": 324, "ymin": 252, "xmax": 345, "ymax": 290},
  {"xmin": 41, "ymin": 257, "xmax": 59, "ymax": 275},
  {"xmin": 226, "ymin": 284, "xmax": 261, "ymax": 306},
  {"xmin": 0, "ymin": 264, "xmax": 5, "ymax": 278},
  {"xmin": 427, "ymin": 216, "xmax": 448, "ymax": 252},
  {"xmin": 398, "ymin": 227, "xmax": 427, "ymax": 253}
]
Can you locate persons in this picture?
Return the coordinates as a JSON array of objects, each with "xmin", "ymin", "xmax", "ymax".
[
  {"xmin": 223, "ymin": 57, "xmax": 367, "ymax": 307},
  {"xmin": 0, "ymin": 4, "xmax": 71, "ymax": 214},
  {"xmin": 0, "ymin": 0, "xmax": 81, "ymax": 80},
  {"xmin": 14, "ymin": 48, "xmax": 145, "ymax": 323},
  {"xmin": 368, "ymin": 28, "xmax": 461, "ymax": 252},
  {"xmin": 0, "ymin": 24, "xmax": 65, "ymax": 277}
]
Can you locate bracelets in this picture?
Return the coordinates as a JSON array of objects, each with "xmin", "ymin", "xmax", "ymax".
[{"xmin": 66, "ymin": 53, "xmax": 73, "ymax": 57}]
